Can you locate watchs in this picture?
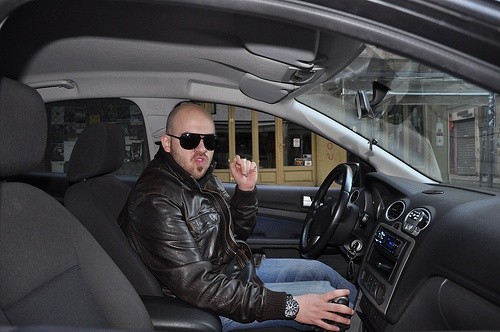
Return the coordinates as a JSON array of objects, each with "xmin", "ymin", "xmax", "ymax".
[{"xmin": 284, "ymin": 292, "xmax": 299, "ymax": 320}]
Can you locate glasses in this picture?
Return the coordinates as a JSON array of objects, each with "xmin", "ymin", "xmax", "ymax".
[{"xmin": 165, "ymin": 130, "xmax": 220, "ymax": 152}]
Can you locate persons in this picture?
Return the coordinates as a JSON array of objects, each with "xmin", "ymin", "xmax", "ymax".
[{"xmin": 116, "ymin": 98, "xmax": 370, "ymax": 332}]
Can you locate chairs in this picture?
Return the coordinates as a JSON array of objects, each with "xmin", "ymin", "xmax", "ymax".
[
  {"xmin": 63, "ymin": 123, "xmax": 223, "ymax": 331},
  {"xmin": 0, "ymin": 76, "xmax": 155, "ymax": 332}
]
